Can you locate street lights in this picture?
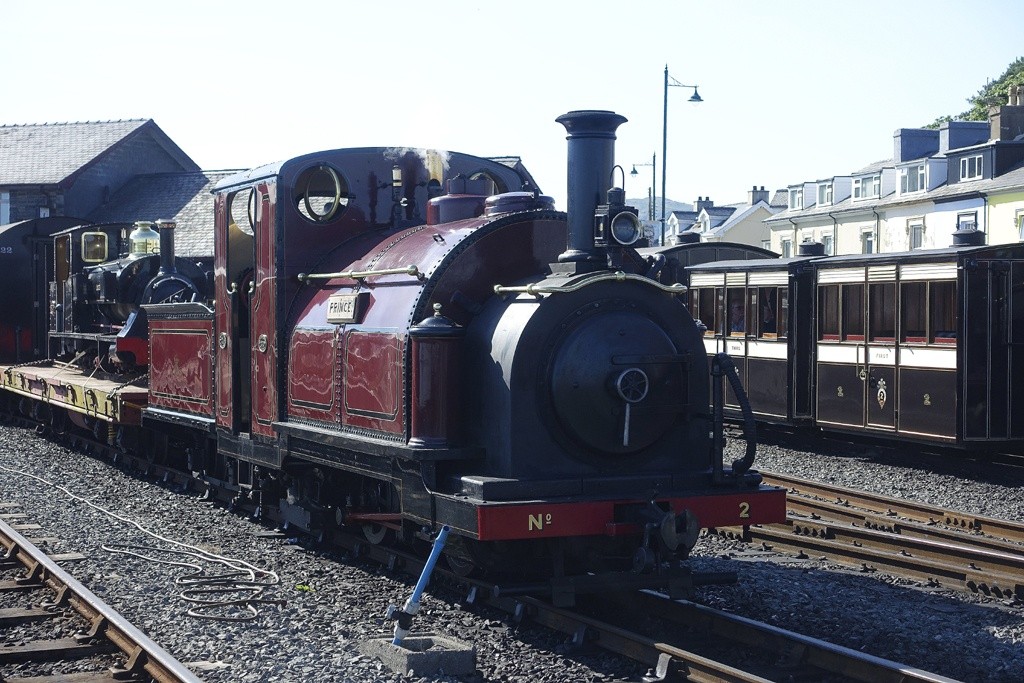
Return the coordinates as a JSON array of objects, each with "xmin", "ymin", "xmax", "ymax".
[
  {"xmin": 662, "ymin": 64, "xmax": 705, "ymax": 245},
  {"xmin": 630, "ymin": 151, "xmax": 655, "ymax": 221}
]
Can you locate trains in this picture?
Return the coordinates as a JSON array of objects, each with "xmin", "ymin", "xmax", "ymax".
[
  {"xmin": 1, "ymin": 108, "xmax": 788, "ymax": 602},
  {"xmin": 634, "ymin": 226, "xmax": 1023, "ymax": 459}
]
